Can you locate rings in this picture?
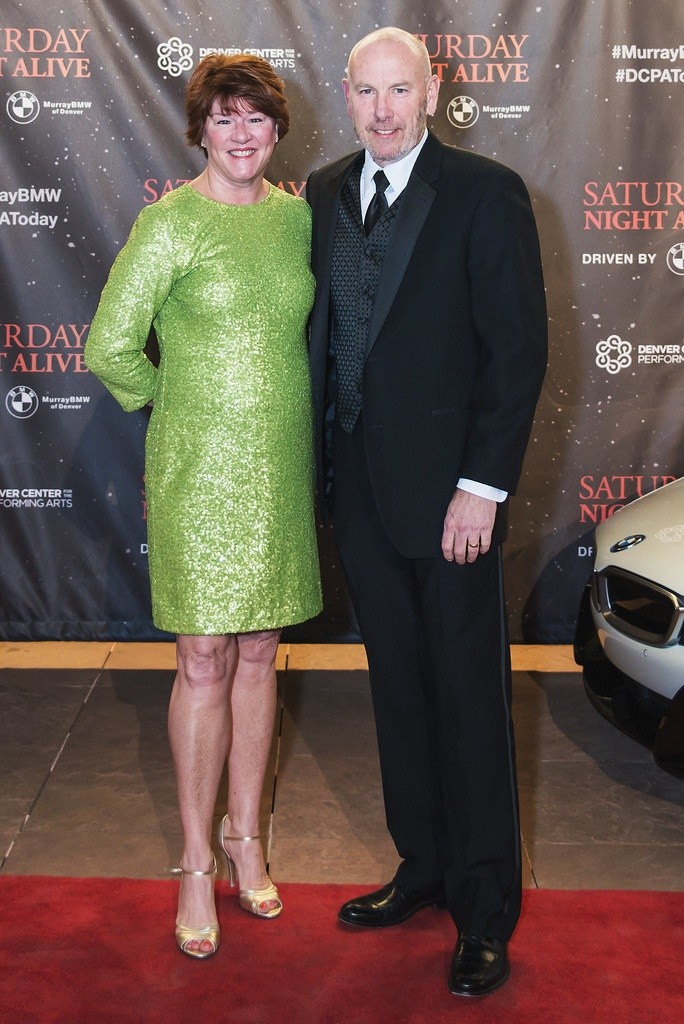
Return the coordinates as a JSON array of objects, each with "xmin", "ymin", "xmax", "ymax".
[{"xmin": 468, "ymin": 542, "xmax": 479, "ymax": 549}]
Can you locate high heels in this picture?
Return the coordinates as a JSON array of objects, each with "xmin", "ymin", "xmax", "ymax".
[
  {"xmin": 171, "ymin": 851, "xmax": 220, "ymax": 959},
  {"xmin": 217, "ymin": 813, "xmax": 283, "ymax": 919}
]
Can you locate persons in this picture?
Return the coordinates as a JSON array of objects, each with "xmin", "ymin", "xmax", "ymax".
[
  {"xmin": 85, "ymin": 52, "xmax": 325, "ymax": 960},
  {"xmin": 311, "ymin": 28, "xmax": 551, "ymax": 998}
]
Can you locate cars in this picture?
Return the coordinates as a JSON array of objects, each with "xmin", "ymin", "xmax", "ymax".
[{"xmin": 572, "ymin": 476, "xmax": 683, "ymax": 781}]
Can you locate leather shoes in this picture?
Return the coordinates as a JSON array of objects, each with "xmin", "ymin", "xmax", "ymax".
[
  {"xmin": 337, "ymin": 883, "xmax": 447, "ymax": 928},
  {"xmin": 448, "ymin": 933, "xmax": 510, "ymax": 996}
]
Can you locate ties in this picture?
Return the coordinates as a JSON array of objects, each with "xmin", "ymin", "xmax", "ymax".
[{"xmin": 364, "ymin": 170, "xmax": 390, "ymax": 238}]
